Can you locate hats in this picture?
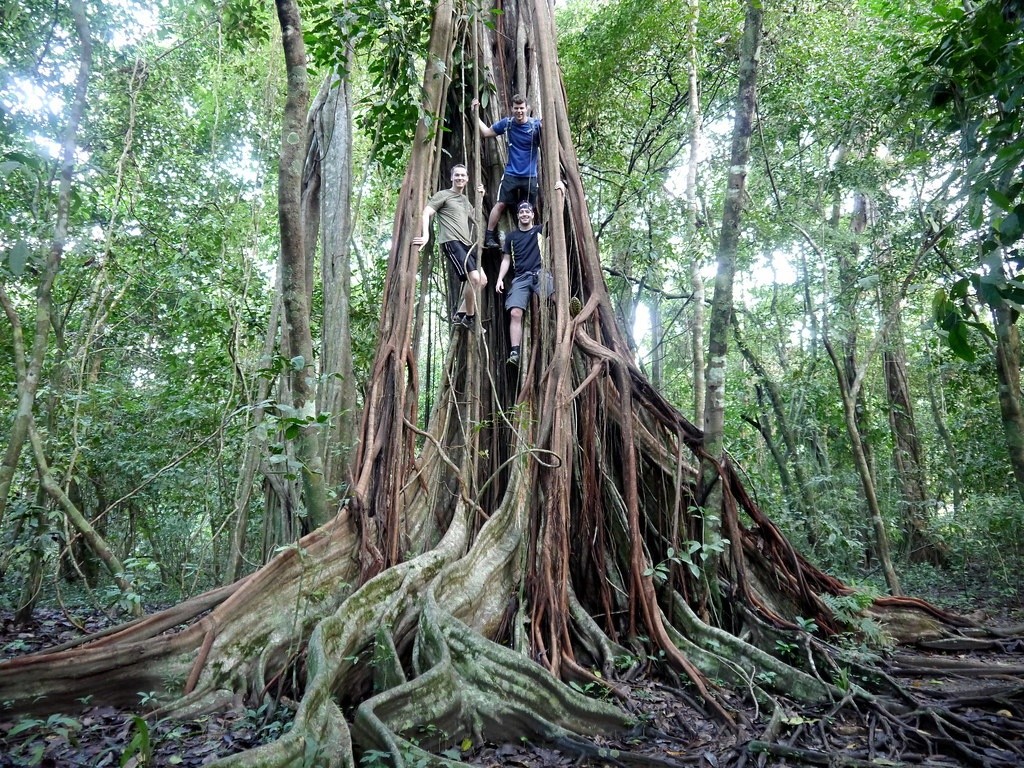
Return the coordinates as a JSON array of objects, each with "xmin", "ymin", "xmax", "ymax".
[{"xmin": 517, "ymin": 200, "xmax": 534, "ymax": 213}]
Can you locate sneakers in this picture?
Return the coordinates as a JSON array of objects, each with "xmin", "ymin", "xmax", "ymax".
[
  {"xmin": 454, "ymin": 314, "xmax": 462, "ymax": 326},
  {"xmin": 505, "ymin": 350, "xmax": 519, "ymax": 368},
  {"xmin": 461, "ymin": 315, "xmax": 487, "ymax": 335}
]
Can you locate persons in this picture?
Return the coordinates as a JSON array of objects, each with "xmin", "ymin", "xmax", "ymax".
[
  {"xmin": 413, "ymin": 163, "xmax": 487, "ymax": 333},
  {"xmin": 495, "ymin": 182, "xmax": 569, "ymax": 364},
  {"xmin": 473, "ymin": 96, "xmax": 545, "ymax": 247}
]
